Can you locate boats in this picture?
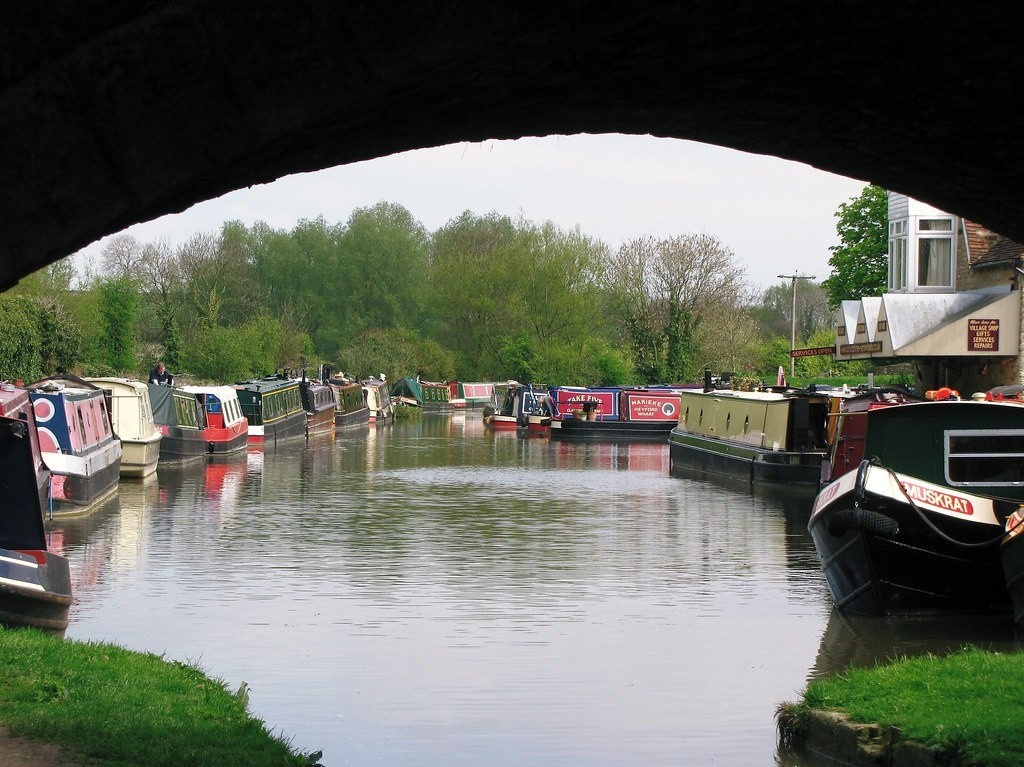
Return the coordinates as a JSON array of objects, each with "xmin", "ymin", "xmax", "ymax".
[
  {"xmin": 668, "ymin": 368, "xmax": 839, "ymax": 483},
  {"xmin": 81, "ymin": 377, "xmax": 163, "ymax": 478},
  {"xmin": 176, "ymin": 385, "xmax": 248, "ymax": 455},
  {"xmin": 807, "ymin": 382, "xmax": 1024, "ymax": 625},
  {"xmin": 234, "ymin": 362, "xmax": 549, "ymax": 449},
  {"xmin": 0, "ymin": 367, "xmax": 122, "ymax": 633},
  {"xmin": 145, "ymin": 382, "xmax": 207, "ymax": 466},
  {"xmin": 542, "ymin": 385, "xmax": 716, "ymax": 439}
]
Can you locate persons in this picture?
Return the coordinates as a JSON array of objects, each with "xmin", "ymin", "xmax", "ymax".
[{"xmin": 148, "ymin": 362, "xmax": 174, "ymax": 388}]
